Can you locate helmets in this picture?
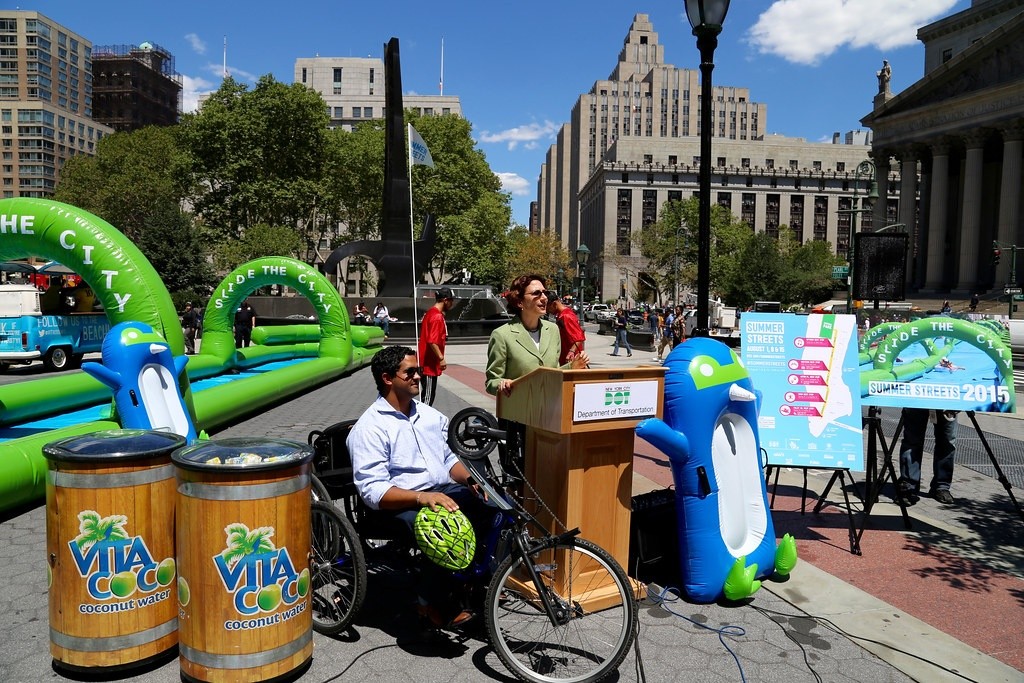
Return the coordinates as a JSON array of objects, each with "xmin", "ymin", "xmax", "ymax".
[{"xmin": 414, "ymin": 504, "xmax": 476, "ymax": 570}]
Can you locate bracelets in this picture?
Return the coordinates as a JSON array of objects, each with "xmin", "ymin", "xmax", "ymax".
[{"xmin": 416, "ymin": 490, "xmax": 425, "ymax": 503}]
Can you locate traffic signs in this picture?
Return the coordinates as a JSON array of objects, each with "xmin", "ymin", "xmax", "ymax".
[{"xmin": 1003, "ymin": 283, "xmax": 1021, "ymax": 295}]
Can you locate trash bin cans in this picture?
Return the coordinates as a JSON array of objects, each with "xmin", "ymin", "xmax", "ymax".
[
  {"xmin": 170, "ymin": 436, "xmax": 315, "ymax": 683},
  {"xmin": 39, "ymin": 428, "xmax": 187, "ymax": 683},
  {"xmin": 628, "ymin": 484, "xmax": 680, "ymax": 586}
]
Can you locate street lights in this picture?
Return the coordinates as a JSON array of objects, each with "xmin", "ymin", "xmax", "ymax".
[
  {"xmin": 557, "ymin": 268, "xmax": 564, "ymax": 300},
  {"xmin": 685, "ymin": 0, "xmax": 730, "ymax": 340},
  {"xmin": 846, "ymin": 160, "xmax": 880, "ymax": 315},
  {"xmin": 576, "ymin": 241, "xmax": 590, "ymax": 332},
  {"xmin": 674, "ymin": 226, "xmax": 688, "ymax": 318}
]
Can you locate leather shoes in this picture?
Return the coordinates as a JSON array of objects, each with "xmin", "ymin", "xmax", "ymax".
[
  {"xmin": 462, "ymin": 582, "xmax": 507, "ymax": 600},
  {"xmin": 417, "ymin": 603, "xmax": 472, "ymax": 626}
]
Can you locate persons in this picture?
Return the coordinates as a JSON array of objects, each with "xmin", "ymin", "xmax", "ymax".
[
  {"xmin": 545, "ymin": 291, "xmax": 585, "ymax": 365},
  {"xmin": 354, "ymin": 301, "xmax": 398, "ymax": 340},
  {"xmin": 971, "ymin": 294, "xmax": 979, "ymax": 311},
  {"xmin": 181, "ymin": 301, "xmax": 198, "ymax": 355},
  {"xmin": 345, "ymin": 344, "xmax": 508, "ymax": 626},
  {"xmin": 486, "ymin": 274, "xmax": 589, "ymax": 488},
  {"xmin": 234, "ymin": 296, "xmax": 257, "ymax": 349},
  {"xmin": 609, "ymin": 302, "xmax": 688, "ymax": 360},
  {"xmin": 893, "ymin": 310, "xmax": 958, "ymax": 508},
  {"xmin": 865, "ymin": 318, "xmax": 870, "ymax": 331},
  {"xmin": 414, "ymin": 286, "xmax": 458, "ymax": 407}
]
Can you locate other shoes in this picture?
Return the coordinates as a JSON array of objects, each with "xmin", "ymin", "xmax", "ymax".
[
  {"xmin": 627, "ymin": 353, "xmax": 631, "ymax": 357},
  {"xmin": 610, "ymin": 353, "xmax": 616, "ymax": 356},
  {"xmin": 929, "ymin": 488, "xmax": 955, "ymax": 503},
  {"xmin": 653, "ymin": 357, "xmax": 661, "ymax": 361},
  {"xmin": 894, "ymin": 491, "xmax": 917, "ymax": 505}
]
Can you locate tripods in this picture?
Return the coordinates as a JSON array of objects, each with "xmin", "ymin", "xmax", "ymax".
[{"xmin": 812, "ymin": 301, "xmax": 913, "ymax": 531}]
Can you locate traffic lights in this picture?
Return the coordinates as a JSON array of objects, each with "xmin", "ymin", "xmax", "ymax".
[{"xmin": 995, "ymin": 251, "xmax": 1002, "ymax": 266}]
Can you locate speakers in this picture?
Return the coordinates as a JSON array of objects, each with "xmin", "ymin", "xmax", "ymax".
[{"xmin": 852, "ymin": 232, "xmax": 908, "ymax": 302}]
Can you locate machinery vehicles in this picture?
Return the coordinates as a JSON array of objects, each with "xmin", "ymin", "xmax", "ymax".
[{"xmin": 683, "ymin": 292, "xmax": 741, "ymax": 346}]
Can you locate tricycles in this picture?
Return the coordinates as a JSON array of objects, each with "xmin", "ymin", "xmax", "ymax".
[{"xmin": 308, "ymin": 406, "xmax": 639, "ymax": 683}]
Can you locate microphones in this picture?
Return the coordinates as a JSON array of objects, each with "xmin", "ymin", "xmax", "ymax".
[{"xmin": 557, "ymin": 319, "xmax": 591, "ymax": 369}]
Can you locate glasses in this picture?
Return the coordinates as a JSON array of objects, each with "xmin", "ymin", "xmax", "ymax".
[
  {"xmin": 384, "ymin": 366, "xmax": 424, "ymax": 376},
  {"xmin": 523, "ymin": 290, "xmax": 550, "ymax": 297}
]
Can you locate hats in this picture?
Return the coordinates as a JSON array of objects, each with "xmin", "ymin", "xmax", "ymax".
[{"xmin": 435, "ymin": 287, "xmax": 462, "ymax": 299}]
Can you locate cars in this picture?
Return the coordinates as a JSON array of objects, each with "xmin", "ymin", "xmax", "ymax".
[{"xmin": 571, "ymin": 302, "xmax": 644, "ymax": 325}]
